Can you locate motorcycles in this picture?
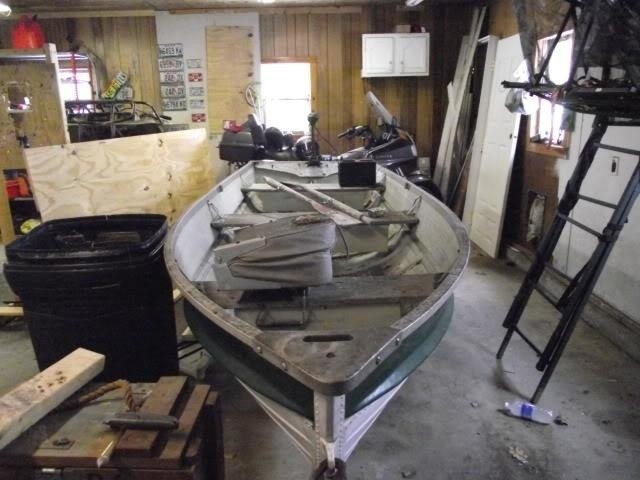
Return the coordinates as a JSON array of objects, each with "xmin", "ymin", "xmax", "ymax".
[{"xmin": 220, "ymin": 93, "xmax": 443, "ymax": 206}]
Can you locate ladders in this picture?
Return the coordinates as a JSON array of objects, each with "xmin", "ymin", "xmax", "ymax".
[{"xmin": 497, "ymin": 96, "xmax": 640, "ymax": 405}]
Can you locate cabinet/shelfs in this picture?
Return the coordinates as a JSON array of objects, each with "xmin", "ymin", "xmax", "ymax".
[
  {"xmin": 0, "ymin": 42, "xmax": 71, "ymax": 317},
  {"xmin": 359, "ymin": 32, "xmax": 430, "ymax": 80}
]
